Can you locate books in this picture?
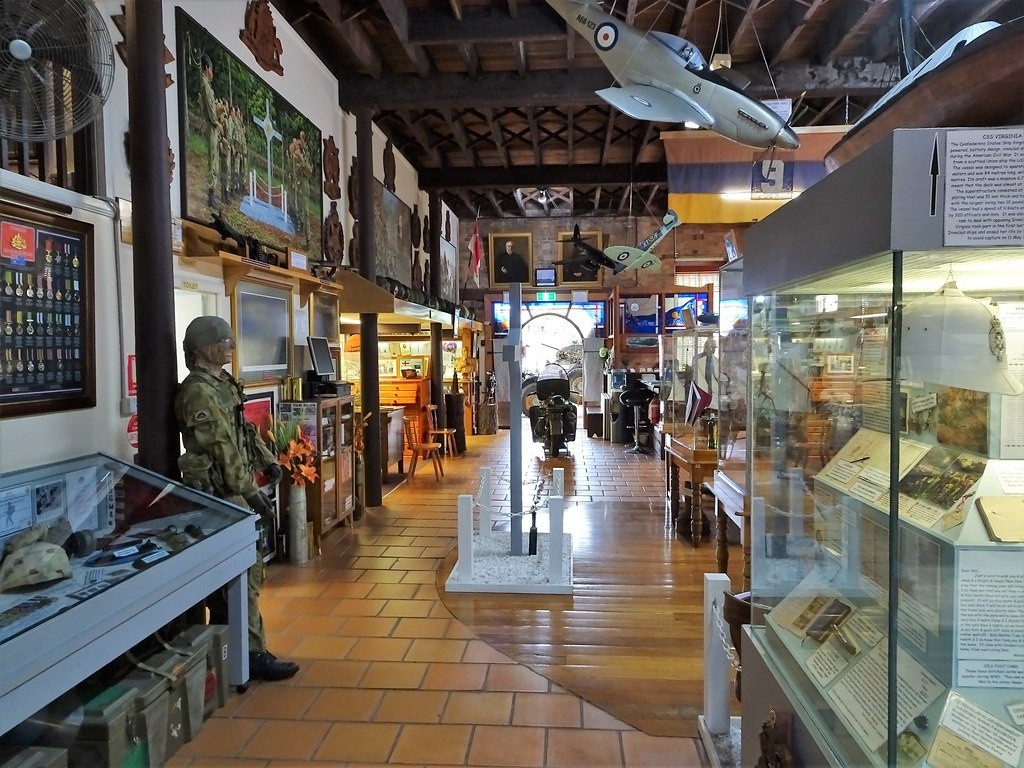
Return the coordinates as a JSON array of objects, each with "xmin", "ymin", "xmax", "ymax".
[{"xmin": 683, "ymin": 381, "xmax": 711, "ymax": 427}]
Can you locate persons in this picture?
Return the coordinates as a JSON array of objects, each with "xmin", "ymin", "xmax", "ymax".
[
  {"xmin": 176, "ymin": 317, "xmax": 300, "ymax": 682},
  {"xmin": 495, "ymin": 240, "xmax": 529, "ymax": 282},
  {"xmin": 197, "ymin": 54, "xmax": 313, "ymax": 254},
  {"xmin": 896, "ymin": 461, "xmax": 978, "ymax": 511}
]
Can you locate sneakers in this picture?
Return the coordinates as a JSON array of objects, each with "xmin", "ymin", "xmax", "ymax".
[{"xmin": 250, "ymin": 650, "xmax": 301, "ymax": 681}]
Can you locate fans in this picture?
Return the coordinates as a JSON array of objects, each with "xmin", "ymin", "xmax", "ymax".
[{"xmin": 0, "ymin": 0, "xmax": 115, "ymax": 143}]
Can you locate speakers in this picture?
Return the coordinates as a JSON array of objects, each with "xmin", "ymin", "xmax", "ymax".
[{"xmin": 303, "ymin": 382, "xmax": 319, "ymax": 399}]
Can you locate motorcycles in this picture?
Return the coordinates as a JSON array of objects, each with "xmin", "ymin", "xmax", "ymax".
[{"xmin": 526, "ymin": 363, "xmax": 578, "ymax": 458}]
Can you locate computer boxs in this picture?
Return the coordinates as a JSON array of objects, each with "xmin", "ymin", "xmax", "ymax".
[{"xmin": 319, "ymin": 384, "xmax": 351, "ymax": 398}]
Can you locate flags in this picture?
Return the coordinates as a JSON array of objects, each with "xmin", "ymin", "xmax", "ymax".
[{"xmin": 466, "ymin": 219, "xmax": 483, "ymax": 289}]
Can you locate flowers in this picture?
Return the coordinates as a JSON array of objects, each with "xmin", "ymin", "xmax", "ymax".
[
  {"xmin": 257, "ymin": 414, "xmax": 320, "ymax": 487},
  {"xmin": 355, "ymin": 412, "xmax": 372, "ymax": 450}
]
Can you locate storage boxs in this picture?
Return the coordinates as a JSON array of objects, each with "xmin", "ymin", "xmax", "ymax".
[{"xmin": 0, "ymin": 625, "xmax": 230, "ymax": 768}]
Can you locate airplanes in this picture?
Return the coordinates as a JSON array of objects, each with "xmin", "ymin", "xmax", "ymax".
[
  {"xmin": 550, "ymin": 2, "xmax": 813, "ymax": 184},
  {"xmin": 550, "ymin": 211, "xmax": 683, "ymax": 283}
]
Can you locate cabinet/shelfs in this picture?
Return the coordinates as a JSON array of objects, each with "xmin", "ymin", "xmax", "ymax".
[{"xmin": 279, "ymin": 395, "xmax": 355, "ymax": 554}]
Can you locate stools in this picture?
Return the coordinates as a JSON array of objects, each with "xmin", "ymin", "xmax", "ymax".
[
  {"xmin": 618, "ymin": 390, "xmax": 653, "ymax": 454},
  {"xmin": 586, "ymin": 406, "xmax": 603, "ymax": 438}
]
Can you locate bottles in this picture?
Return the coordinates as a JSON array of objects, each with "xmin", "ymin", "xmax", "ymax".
[
  {"xmin": 610, "ymin": 368, "xmax": 627, "ymax": 389},
  {"xmin": 693, "ymin": 416, "xmax": 709, "ymax": 450},
  {"xmin": 708, "ymin": 412, "xmax": 719, "ymax": 449}
]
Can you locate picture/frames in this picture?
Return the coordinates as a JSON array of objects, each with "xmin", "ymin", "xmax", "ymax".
[
  {"xmin": 440, "ymin": 235, "xmax": 456, "ymax": 305},
  {"xmin": 253, "ymin": 500, "xmax": 278, "ymax": 567},
  {"xmin": 0, "ymin": 200, "xmax": 97, "ymax": 419},
  {"xmin": 558, "ymin": 231, "xmax": 602, "ymax": 286},
  {"xmin": 174, "ymin": 5, "xmax": 324, "ymax": 264},
  {"xmin": 230, "ymin": 275, "xmax": 295, "ymax": 388},
  {"xmin": 309, "ymin": 290, "xmax": 340, "ymax": 342},
  {"xmin": 243, "ymin": 391, "xmax": 276, "ymax": 500},
  {"xmin": 373, "ymin": 176, "xmax": 413, "ymax": 289},
  {"xmin": 378, "ymin": 356, "xmax": 430, "ymax": 379},
  {"xmin": 489, "ymin": 233, "xmax": 532, "ymax": 287}
]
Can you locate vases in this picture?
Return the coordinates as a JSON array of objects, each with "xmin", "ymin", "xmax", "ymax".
[
  {"xmin": 353, "ymin": 451, "xmax": 365, "ymax": 521},
  {"xmin": 286, "ymin": 484, "xmax": 308, "ymax": 564}
]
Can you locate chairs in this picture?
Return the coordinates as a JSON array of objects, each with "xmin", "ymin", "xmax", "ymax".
[
  {"xmin": 424, "ymin": 404, "xmax": 458, "ymax": 459},
  {"xmin": 403, "ymin": 416, "xmax": 444, "ymax": 483}
]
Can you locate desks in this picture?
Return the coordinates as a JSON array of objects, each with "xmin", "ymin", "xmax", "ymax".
[
  {"xmin": 664, "ymin": 447, "xmax": 773, "ymax": 548},
  {"xmin": 704, "ymin": 481, "xmax": 814, "ymax": 593}
]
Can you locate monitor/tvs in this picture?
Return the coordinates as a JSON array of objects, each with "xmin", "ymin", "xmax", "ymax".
[{"xmin": 307, "ymin": 336, "xmax": 335, "ymax": 376}]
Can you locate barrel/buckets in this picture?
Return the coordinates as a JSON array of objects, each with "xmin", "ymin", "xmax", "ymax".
[{"xmin": 631, "ymin": 432, "xmax": 650, "ymax": 446}]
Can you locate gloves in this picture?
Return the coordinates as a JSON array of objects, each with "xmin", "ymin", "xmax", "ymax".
[
  {"xmin": 267, "ymin": 464, "xmax": 282, "ymax": 489},
  {"xmin": 246, "ymin": 490, "xmax": 277, "ymax": 519}
]
